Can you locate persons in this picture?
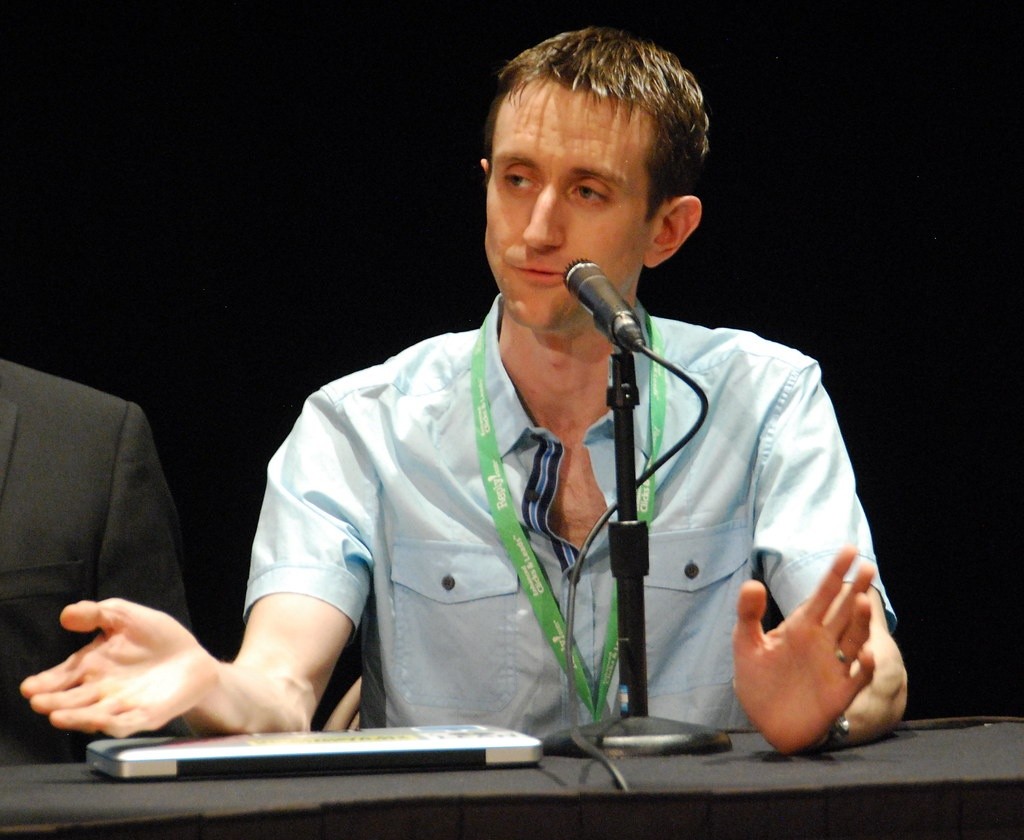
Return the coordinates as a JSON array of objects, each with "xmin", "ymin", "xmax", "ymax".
[
  {"xmin": 0, "ymin": 356, "xmax": 193, "ymax": 766},
  {"xmin": 18, "ymin": 27, "xmax": 909, "ymax": 759}
]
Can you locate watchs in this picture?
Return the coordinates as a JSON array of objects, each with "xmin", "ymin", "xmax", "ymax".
[{"xmin": 821, "ymin": 712, "xmax": 850, "ymax": 748}]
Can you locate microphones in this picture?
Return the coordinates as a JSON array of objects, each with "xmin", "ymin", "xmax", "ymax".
[{"xmin": 561, "ymin": 257, "xmax": 647, "ymax": 352}]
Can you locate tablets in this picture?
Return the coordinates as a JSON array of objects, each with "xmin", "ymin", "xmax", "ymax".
[{"xmin": 89, "ymin": 722, "xmax": 543, "ymax": 783}]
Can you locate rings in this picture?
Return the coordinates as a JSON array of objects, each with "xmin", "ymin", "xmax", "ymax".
[{"xmin": 835, "ymin": 647, "xmax": 848, "ymax": 663}]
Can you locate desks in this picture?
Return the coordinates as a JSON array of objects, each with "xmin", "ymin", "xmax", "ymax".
[{"xmin": 0, "ymin": 720, "xmax": 1024, "ymax": 840}]
